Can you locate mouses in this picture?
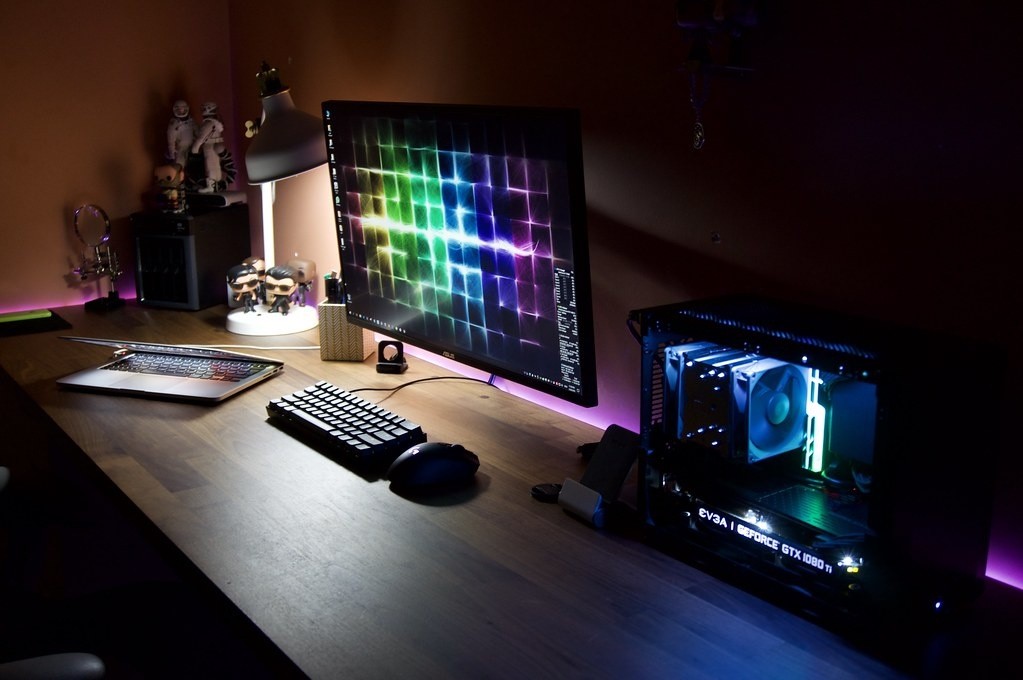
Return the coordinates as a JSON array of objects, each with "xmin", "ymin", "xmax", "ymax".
[{"xmin": 387, "ymin": 442, "xmax": 481, "ymax": 489}]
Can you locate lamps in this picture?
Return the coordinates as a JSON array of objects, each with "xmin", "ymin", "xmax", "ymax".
[{"xmin": 226, "ymin": 62, "xmax": 327, "ymax": 336}]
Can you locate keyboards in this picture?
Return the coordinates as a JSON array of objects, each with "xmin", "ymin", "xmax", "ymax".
[{"xmin": 265, "ymin": 382, "xmax": 427, "ymax": 484}]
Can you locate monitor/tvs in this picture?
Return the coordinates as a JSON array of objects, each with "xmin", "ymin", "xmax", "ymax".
[{"xmin": 320, "ymin": 100, "xmax": 600, "ymax": 408}]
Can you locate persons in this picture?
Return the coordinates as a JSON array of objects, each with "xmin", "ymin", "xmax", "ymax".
[
  {"xmin": 193, "ymin": 102, "xmax": 226, "ymax": 194},
  {"xmin": 167, "ymin": 101, "xmax": 202, "ymax": 177},
  {"xmin": 263, "ymin": 265, "xmax": 296, "ymax": 316},
  {"xmin": 287, "ymin": 258, "xmax": 316, "ymax": 308},
  {"xmin": 227, "ymin": 265, "xmax": 260, "ymax": 313},
  {"xmin": 241, "ymin": 257, "xmax": 266, "ymax": 304}
]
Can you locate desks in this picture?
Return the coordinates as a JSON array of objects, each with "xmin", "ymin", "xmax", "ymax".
[{"xmin": 1, "ymin": 299, "xmax": 1022, "ymax": 680}]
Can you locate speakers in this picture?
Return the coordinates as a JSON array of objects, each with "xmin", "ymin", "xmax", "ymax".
[{"xmin": 130, "ymin": 204, "xmax": 250, "ymax": 311}]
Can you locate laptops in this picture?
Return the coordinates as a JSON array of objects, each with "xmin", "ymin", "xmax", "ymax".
[{"xmin": 55, "ymin": 335, "xmax": 287, "ymax": 402}]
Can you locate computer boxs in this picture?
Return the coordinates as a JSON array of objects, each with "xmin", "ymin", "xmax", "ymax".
[{"xmin": 608, "ymin": 292, "xmax": 998, "ymax": 680}]
